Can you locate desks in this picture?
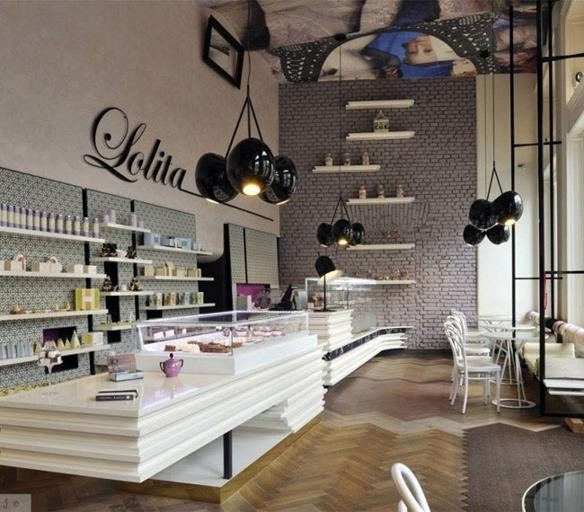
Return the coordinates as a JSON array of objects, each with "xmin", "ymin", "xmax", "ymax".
[{"xmin": 476, "ymin": 315, "xmax": 550, "ymax": 409}]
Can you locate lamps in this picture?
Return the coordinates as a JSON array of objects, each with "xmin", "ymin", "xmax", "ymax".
[
  {"xmin": 313, "ymin": 31, "xmax": 367, "ymax": 312},
  {"xmin": 460, "ymin": 2, "xmax": 525, "ymax": 248},
  {"xmin": 192, "ymin": 0, "xmax": 301, "ymax": 209}
]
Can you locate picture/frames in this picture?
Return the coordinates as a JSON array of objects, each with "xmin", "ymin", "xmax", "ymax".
[{"xmin": 202, "ymin": 14, "xmax": 246, "ymax": 90}]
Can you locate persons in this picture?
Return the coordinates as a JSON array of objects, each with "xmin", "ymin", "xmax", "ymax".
[{"xmin": 403, "ymin": 36, "xmax": 437, "ymax": 65}]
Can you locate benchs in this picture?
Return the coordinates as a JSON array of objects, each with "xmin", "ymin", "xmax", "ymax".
[{"xmin": 515, "ymin": 310, "xmax": 584, "ymax": 397}]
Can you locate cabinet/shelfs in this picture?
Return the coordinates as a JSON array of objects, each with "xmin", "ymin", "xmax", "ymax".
[{"xmin": 0, "ymin": 219, "xmax": 217, "ymax": 370}]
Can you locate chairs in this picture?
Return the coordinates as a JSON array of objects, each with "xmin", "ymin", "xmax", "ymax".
[{"xmin": 444, "ymin": 307, "xmax": 503, "ymax": 415}]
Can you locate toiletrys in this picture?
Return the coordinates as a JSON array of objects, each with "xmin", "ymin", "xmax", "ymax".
[
  {"xmin": 102, "ymin": 214, "xmax": 109, "ymax": 222},
  {"xmin": 109, "ymin": 208, "xmax": 116, "ymax": 224},
  {"xmin": 137, "ymin": 221, "xmax": 144, "ymax": 227},
  {"xmin": 129, "ymin": 213, "xmax": 137, "ymax": 226},
  {"xmin": 0, "ymin": 203, "xmax": 99, "ymax": 238}
]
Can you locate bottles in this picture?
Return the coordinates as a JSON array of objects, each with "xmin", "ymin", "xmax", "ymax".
[
  {"xmin": 326, "ymin": 150, "xmax": 405, "ymax": 199},
  {"xmin": 160, "ymin": 353, "xmax": 183, "ymax": 377},
  {"xmin": 0, "ymin": 203, "xmax": 100, "ymax": 238}
]
